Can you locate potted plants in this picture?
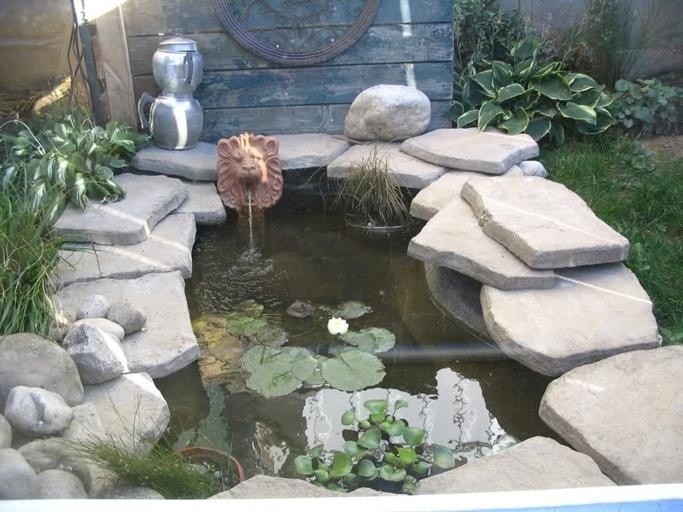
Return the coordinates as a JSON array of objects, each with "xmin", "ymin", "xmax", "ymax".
[
  {"xmin": 305, "ymin": 128, "xmax": 416, "ymax": 235},
  {"xmin": 38, "ymin": 385, "xmax": 245, "ymax": 499}
]
